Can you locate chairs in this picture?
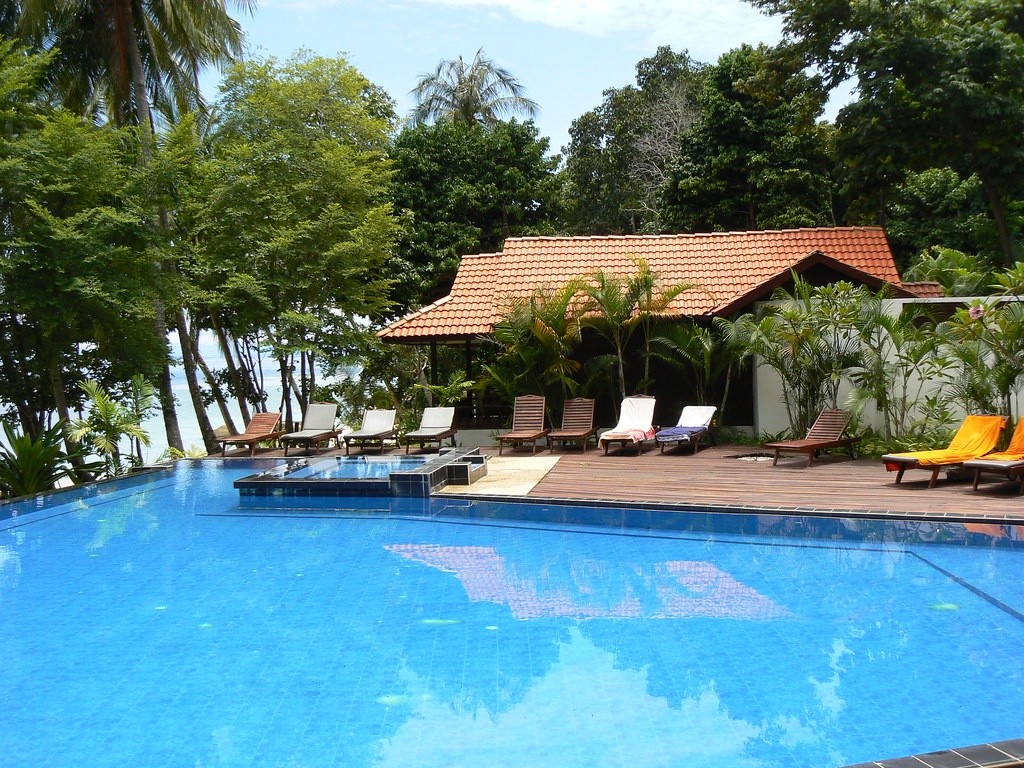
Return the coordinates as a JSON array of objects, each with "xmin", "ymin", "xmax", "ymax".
[
  {"xmin": 881, "ymin": 414, "xmax": 1011, "ymax": 486},
  {"xmin": 656, "ymin": 405, "xmax": 718, "ymax": 455},
  {"xmin": 343, "ymin": 408, "xmax": 401, "ymax": 457},
  {"xmin": 547, "ymin": 397, "xmax": 600, "ymax": 453},
  {"xmin": 963, "ymin": 415, "xmax": 1024, "ymax": 491},
  {"xmin": 280, "ymin": 400, "xmax": 343, "ymax": 456},
  {"xmin": 763, "ymin": 404, "xmax": 862, "ymax": 467},
  {"xmin": 598, "ymin": 393, "xmax": 661, "ymax": 455},
  {"xmin": 216, "ymin": 411, "xmax": 287, "ymax": 454},
  {"xmin": 496, "ymin": 394, "xmax": 550, "ymax": 457},
  {"xmin": 404, "ymin": 407, "xmax": 458, "ymax": 453}
]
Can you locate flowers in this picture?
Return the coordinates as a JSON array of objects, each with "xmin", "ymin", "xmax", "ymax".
[{"xmin": 967, "ymin": 303, "xmax": 985, "ymax": 322}]
